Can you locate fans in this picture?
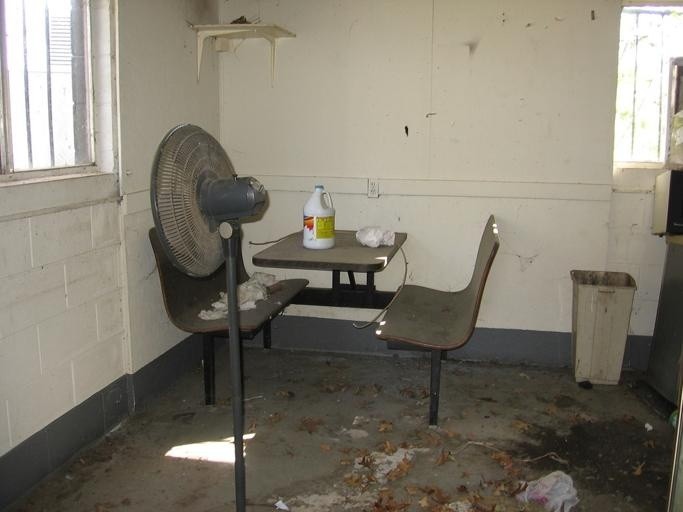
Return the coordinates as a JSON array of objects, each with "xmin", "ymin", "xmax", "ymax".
[{"xmin": 150, "ymin": 122, "xmax": 268, "ymax": 512}]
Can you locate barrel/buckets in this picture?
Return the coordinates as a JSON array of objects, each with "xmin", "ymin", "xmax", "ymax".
[{"xmin": 300, "ymin": 183, "xmax": 338, "ymax": 251}]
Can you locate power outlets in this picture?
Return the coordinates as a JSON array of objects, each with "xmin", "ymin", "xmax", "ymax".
[{"xmin": 367, "ymin": 178, "xmax": 380, "ymax": 199}]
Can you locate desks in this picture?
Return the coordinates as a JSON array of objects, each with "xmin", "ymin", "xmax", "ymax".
[{"xmin": 252, "ymin": 226, "xmax": 408, "ymax": 306}]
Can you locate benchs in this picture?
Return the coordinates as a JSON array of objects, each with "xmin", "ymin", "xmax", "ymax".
[
  {"xmin": 149, "ymin": 226, "xmax": 310, "ymax": 406},
  {"xmin": 374, "ymin": 213, "xmax": 501, "ymax": 429}
]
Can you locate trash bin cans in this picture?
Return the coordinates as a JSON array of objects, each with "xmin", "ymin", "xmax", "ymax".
[{"xmin": 571, "ymin": 270, "xmax": 637, "ymax": 385}]
